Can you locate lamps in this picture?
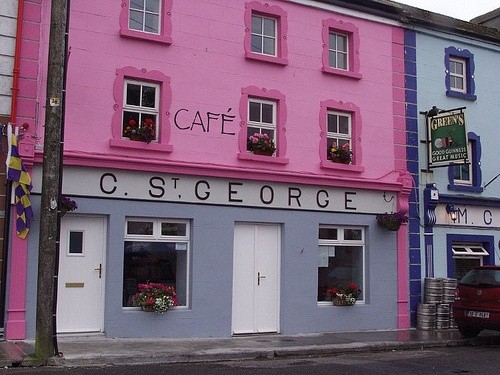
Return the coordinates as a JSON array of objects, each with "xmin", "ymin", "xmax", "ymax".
[{"xmin": 446, "ymin": 200, "xmax": 458, "ymax": 223}]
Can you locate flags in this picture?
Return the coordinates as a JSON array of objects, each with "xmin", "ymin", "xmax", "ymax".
[{"xmin": 6, "ymin": 134, "xmax": 32, "ymax": 239}]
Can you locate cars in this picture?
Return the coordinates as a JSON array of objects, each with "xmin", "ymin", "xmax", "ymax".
[{"xmin": 453, "ymin": 266, "xmax": 500, "ymax": 338}]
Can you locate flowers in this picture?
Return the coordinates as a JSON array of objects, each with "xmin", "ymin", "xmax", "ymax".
[
  {"xmin": 376, "ymin": 209, "xmax": 409, "ymax": 226},
  {"xmin": 329, "ymin": 142, "xmax": 350, "ymax": 159},
  {"xmin": 62, "ymin": 194, "xmax": 80, "ymax": 212},
  {"xmin": 327, "ymin": 281, "xmax": 358, "ymax": 304},
  {"xmin": 125, "ymin": 119, "xmax": 156, "ymax": 140},
  {"xmin": 249, "ymin": 133, "xmax": 277, "ymax": 152},
  {"xmin": 131, "ymin": 283, "xmax": 178, "ymax": 314}
]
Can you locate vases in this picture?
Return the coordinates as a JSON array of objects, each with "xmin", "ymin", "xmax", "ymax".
[
  {"xmin": 332, "ymin": 294, "xmax": 357, "ymax": 306},
  {"xmin": 386, "ymin": 221, "xmax": 401, "ymax": 231},
  {"xmin": 130, "ymin": 134, "xmax": 152, "ymax": 143},
  {"xmin": 141, "ymin": 303, "xmax": 156, "ymax": 312},
  {"xmin": 60, "ymin": 208, "xmax": 67, "ymax": 217},
  {"xmin": 333, "ymin": 156, "xmax": 351, "ymax": 164},
  {"xmin": 253, "ymin": 148, "xmax": 273, "ymax": 156}
]
